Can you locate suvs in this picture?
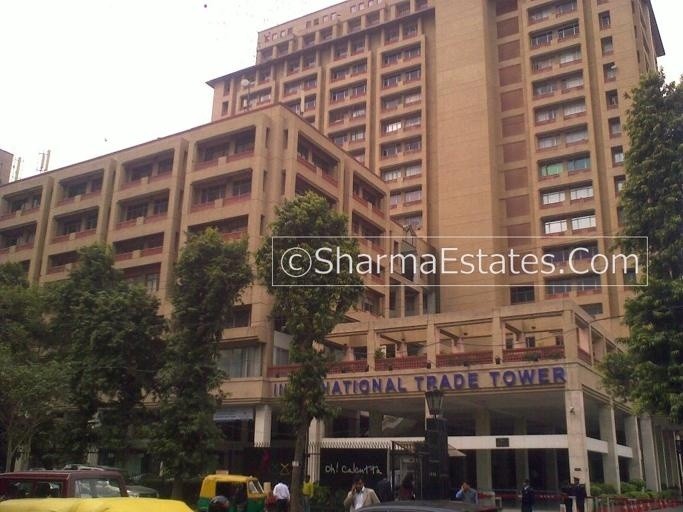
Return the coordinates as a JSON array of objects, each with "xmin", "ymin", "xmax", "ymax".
[{"xmin": 59, "ymin": 464, "xmax": 160, "ymax": 499}]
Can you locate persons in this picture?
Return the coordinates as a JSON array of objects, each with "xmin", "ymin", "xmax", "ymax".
[
  {"xmin": 341, "ymin": 472, "xmax": 382, "ymax": 512},
  {"xmin": 565, "ymin": 476, "xmax": 587, "ymax": 512},
  {"xmin": 520, "ymin": 478, "xmax": 533, "ymax": 511},
  {"xmin": 454, "ymin": 479, "xmax": 479, "ymax": 504},
  {"xmin": 301, "ymin": 474, "xmax": 315, "ymax": 511},
  {"xmin": 272, "ymin": 477, "xmax": 290, "ymax": 511},
  {"xmin": 394, "ymin": 472, "xmax": 417, "ymax": 499}
]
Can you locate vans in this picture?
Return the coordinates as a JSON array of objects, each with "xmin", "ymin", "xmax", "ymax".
[{"xmin": 0, "ymin": 471, "xmax": 128, "ymax": 502}]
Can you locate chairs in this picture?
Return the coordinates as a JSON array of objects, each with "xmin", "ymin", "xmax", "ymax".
[
  {"xmin": 36, "ymin": 482, "xmax": 49, "ymax": 496},
  {"xmin": 51, "ymin": 487, "xmax": 59, "ymax": 496},
  {"xmin": 5, "ymin": 484, "xmax": 22, "ymax": 499}
]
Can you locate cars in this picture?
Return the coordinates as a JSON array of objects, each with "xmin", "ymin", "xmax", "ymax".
[{"xmin": 20, "ymin": 468, "xmax": 59, "ymax": 495}]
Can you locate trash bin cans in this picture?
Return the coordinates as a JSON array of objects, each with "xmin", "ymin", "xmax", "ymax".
[{"xmin": 478, "ymin": 491, "xmax": 495, "ymax": 512}]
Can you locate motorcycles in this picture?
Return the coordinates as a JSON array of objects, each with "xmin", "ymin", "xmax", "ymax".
[{"xmin": 196, "ymin": 473, "xmax": 266, "ymax": 512}]
